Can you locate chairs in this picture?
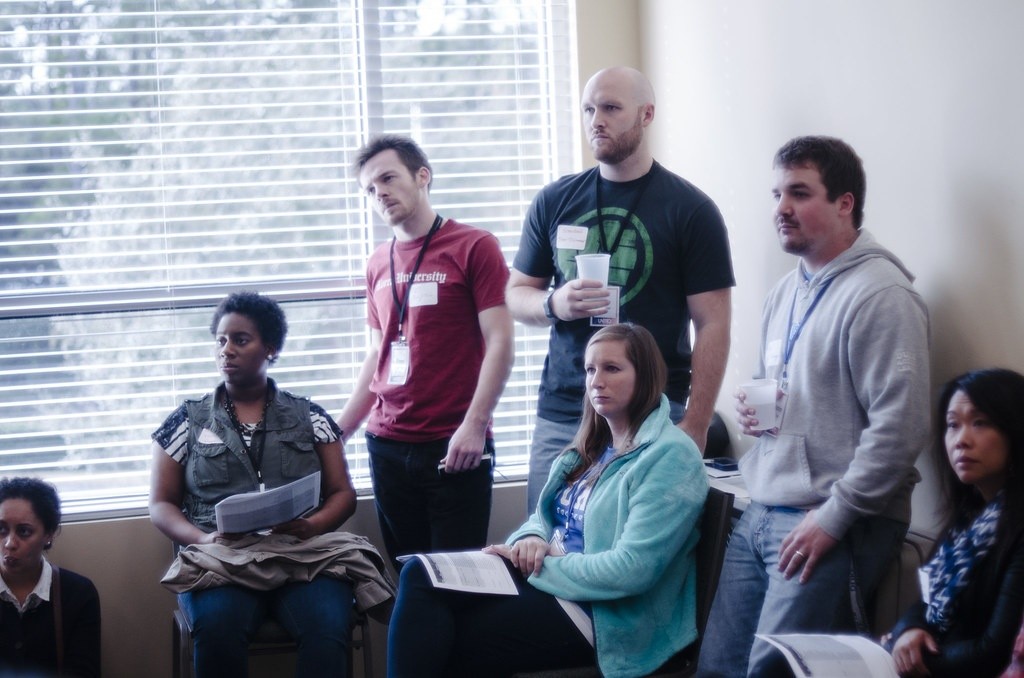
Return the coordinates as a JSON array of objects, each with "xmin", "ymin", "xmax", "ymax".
[
  {"xmin": 512, "ymin": 485, "xmax": 735, "ymax": 678},
  {"xmin": 171, "ymin": 540, "xmax": 375, "ymax": 678}
]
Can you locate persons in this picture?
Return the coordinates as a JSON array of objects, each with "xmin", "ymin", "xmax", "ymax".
[
  {"xmin": 505, "ymin": 63, "xmax": 737, "ymax": 520},
  {"xmin": 149, "ymin": 291, "xmax": 374, "ymax": 677},
  {"xmin": 874, "ymin": 367, "xmax": 1024, "ymax": 678},
  {"xmin": 377, "ymin": 321, "xmax": 709, "ymax": 678},
  {"xmin": 333, "ymin": 135, "xmax": 514, "ymax": 582},
  {"xmin": 0, "ymin": 477, "xmax": 102, "ymax": 678},
  {"xmin": 689, "ymin": 136, "xmax": 931, "ymax": 678}
]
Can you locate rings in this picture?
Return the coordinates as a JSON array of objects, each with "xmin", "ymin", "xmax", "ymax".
[{"xmin": 796, "ymin": 551, "xmax": 806, "ymax": 559}]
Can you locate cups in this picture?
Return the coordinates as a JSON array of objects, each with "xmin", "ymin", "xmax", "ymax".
[
  {"xmin": 575, "ymin": 253, "xmax": 611, "ymax": 310},
  {"xmin": 739, "ymin": 379, "xmax": 777, "ymax": 431}
]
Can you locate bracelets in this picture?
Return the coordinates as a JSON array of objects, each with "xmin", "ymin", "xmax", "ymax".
[{"xmin": 543, "ymin": 289, "xmax": 563, "ymax": 326}]
